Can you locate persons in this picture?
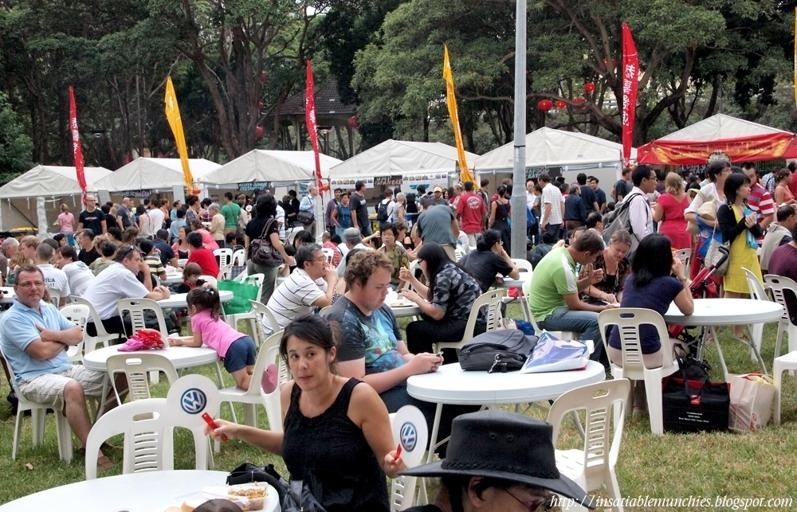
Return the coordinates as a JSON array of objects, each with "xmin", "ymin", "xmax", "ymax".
[
  {"xmin": 199, "ymin": 311, "xmax": 406, "ymax": 512},
  {"xmin": 393, "ymin": 405, "xmax": 559, "ymax": 512},
  {"xmin": 0, "ymin": 148, "xmax": 797, "ymax": 474}
]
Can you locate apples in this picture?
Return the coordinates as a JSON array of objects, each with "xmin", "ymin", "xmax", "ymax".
[{"xmin": 508, "ymin": 287, "xmax": 519, "ymax": 298}]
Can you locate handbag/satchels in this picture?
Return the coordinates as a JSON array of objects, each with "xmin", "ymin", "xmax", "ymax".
[
  {"xmin": 454, "ymin": 326, "xmax": 541, "ymax": 374},
  {"xmin": 249, "ymin": 238, "xmax": 286, "ymax": 269},
  {"xmin": 660, "ymin": 374, "xmax": 732, "ymax": 436},
  {"xmin": 215, "ymin": 274, "xmax": 262, "ymax": 315},
  {"xmin": 600, "ymin": 192, "xmax": 650, "ymax": 261},
  {"xmin": 519, "ymin": 328, "xmax": 598, "ymax": 375},
  {"xmin": 296, "ymin": 209, "xmax": 315, "ymax": 226},
  {"xmin": 703, "ymin": 238, "xmax": 732, "ymax": 275},
  {"xmin": 505, "ymin": 205, "xmax": 537, "ymax": 232},
  {"xmin": 723, "ymin": 368, "xmax": 777, "ymax": 436},
  {"xmin": 682, "ymin": 187, "xmax": 720, "ymax": 235}
]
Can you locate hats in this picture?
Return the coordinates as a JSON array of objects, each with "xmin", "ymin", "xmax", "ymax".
[
  {"xmin": 342, "ymin": 226, "xmax": 361, "ymax": 241},
  {"xmin": 433, "ymin": 186, "xmax": 443, "ymax": 193},
  {"xmin": 392, "ymin": 407, "xmax": 598, "ymax": 511}
]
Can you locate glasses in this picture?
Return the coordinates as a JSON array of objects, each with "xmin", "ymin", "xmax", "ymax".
[
  {"xmin": 122, "ymin": 244, "xmax": 134, "ymax": 258},
  {"xmin": 500, "ymin": 241, "xmax": 504, "ymax": 246},
  {"xmin": 495, "ymin": 483, "xmax": 561, "ymax": 512}
]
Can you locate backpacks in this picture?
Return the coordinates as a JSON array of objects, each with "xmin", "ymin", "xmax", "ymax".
[
  {"xmin": 224, "ymin": 460, "xmax": 330, "ymax": 512},
  {"xmin": 672, "ymin": 342, "xmax": 713, "ymax": 381},
  {"xmin": 376, "ymin": 199, "xmax": 393, "ymax": 224}
]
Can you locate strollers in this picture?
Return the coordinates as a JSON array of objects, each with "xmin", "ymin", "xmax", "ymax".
[{"xmin": 667, "ymin": 244, "xmax": 734, "ymax": 365}]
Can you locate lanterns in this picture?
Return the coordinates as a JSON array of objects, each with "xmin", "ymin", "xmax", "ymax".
[
  {"xmin": 535, "ymin": 97, "xmax": 552, "ymax": 112},
  {"xmin": 259, "ymin": 70, "xmax": 272, "ymax": 83},
  {"xmin": 258, "ymin": 101, "xmax": 263, "ymax": 110},
  {"xmin": 256, "ymin": 126, "xmax": 263, "ymax": 139},
  {"xmin": 346, "ymin": 115, "xmax": 358, "ymax": 127},
  {"xmin": 603, "ymin": 58, "xmax": 616, "ymax": 71},
  {"xmin": 555, "ymin": 100, "xmax": 565, "ymax": 109},
  {"xmin": 583, "ymin": 83, "xmax": 594, "ymax": 92},
  {"xmin": 572, "ymin": 98, "xmax": 586, "ymax": 110}
]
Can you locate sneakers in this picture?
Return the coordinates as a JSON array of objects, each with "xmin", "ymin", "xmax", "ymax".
[
  {"xmin": 631, "ymin": 405, "xmax": 648, "ymax": 419},
  {"xmin": 260, "ymin": 363, "xmax": 278, "ymax": 395},
  {"xmin": 95, "ymin": 455, "xmax": 115, "ymax": 473},
  {"xmin": 80, "ymin": 441, "xmax": 124, "ymax": 456}
]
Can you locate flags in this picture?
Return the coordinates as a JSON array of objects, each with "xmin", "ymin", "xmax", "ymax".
[
  {"xmin": 66, "ymin": 86, "xmax": 93, "ymax": 196},
  {"xmin": 304, "ymin": 58, "xmax": 327, "ymax": 187},
  {"xmin": 439, "ymin": 41, "xmax": 476, "ymax": 189},
  {"xmin": 160, "ymin": 75, "xmax": 197, "ymax": 191},
  {"xmin": 618, "ymin": 22, "xmax": 641, "ymax": 163}
]
